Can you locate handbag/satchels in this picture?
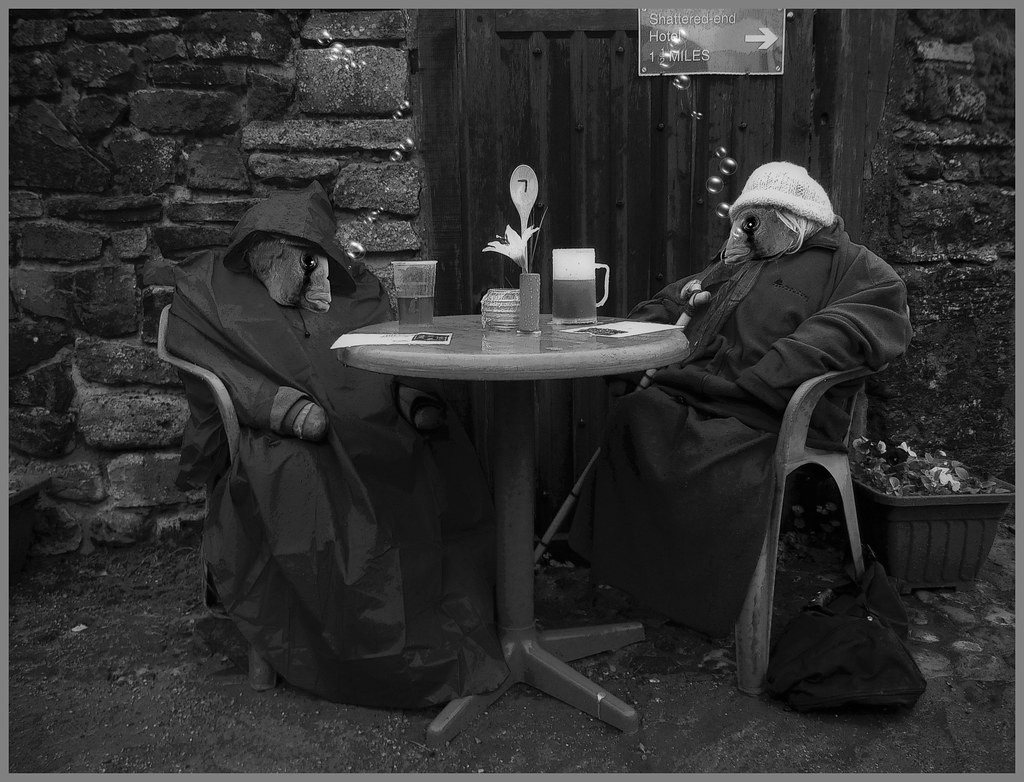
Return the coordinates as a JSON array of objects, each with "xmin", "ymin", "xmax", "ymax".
[{"xmin": 767, "ymin": 604, "xmax": 926, "ymax": 712}]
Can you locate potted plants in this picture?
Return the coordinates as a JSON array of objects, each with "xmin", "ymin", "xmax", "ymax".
[{"xmin": 846, "ymin": 434, "xmax": 1016, "ymax": 593}]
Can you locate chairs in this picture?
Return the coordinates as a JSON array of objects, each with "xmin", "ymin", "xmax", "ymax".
[
  {"xmin": 158, "ymin": 303, "xmax": 280, "ymax": 691},
  {"xmin": 734, "ymin": 304, "xmax": 909, "ymax": 703}
]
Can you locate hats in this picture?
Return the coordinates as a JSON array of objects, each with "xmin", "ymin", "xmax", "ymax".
[{"xmin": 728, "ymin": 162, "xmax": 834, "ymax": 227}]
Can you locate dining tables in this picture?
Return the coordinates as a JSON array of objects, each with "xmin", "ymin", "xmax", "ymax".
[{"xmin": 335, "ymin": 310, "xmax": 691, "ymax": 748}]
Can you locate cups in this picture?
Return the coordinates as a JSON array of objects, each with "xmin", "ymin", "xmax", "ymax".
[
  {"xmin": 391, "ymin": 259, "xmax": 437, "ymax": 327},
  {"xmin": 551, "ymin": 247, "xmax": 610, "ymax": 324},
  {"xmin": 480, "ymin": 286, "xmax": 521, "ymax": 332}
]
